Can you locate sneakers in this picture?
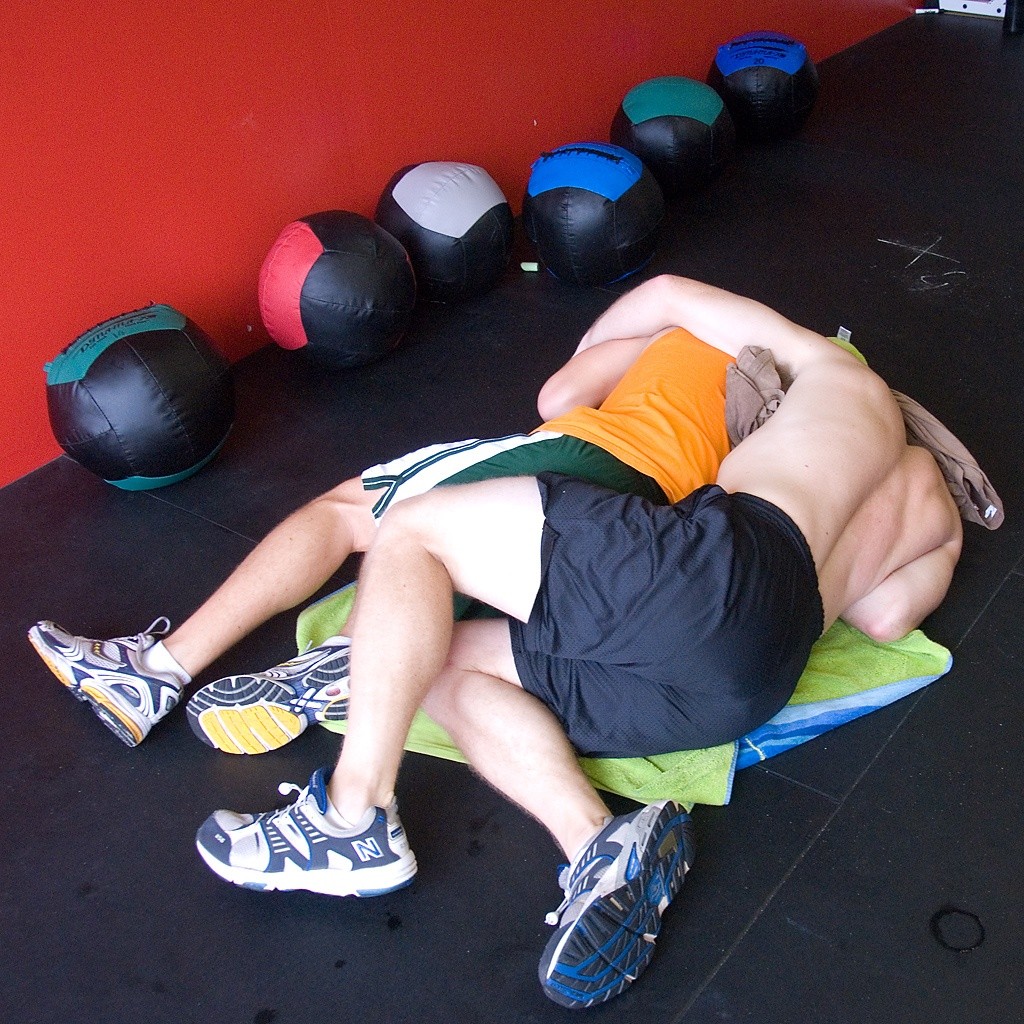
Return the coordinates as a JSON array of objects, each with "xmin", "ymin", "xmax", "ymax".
[
  {"xmin": 27, "ymin": 616, "xmax": 185, "ymax": 745},
  {"xmin": 537, "ymin": 800, "xmax": 699, "ymax": 1010},
  {"xmin": 185, "ymin": 634, "xmax": 355, "ymax": 756},
  {"xmin": 196, "ymin": 766, "xmax": 416, "ymax": 897}
]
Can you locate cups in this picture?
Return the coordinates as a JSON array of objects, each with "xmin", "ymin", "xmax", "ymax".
[{"xmin": 1002, "ymin": 0, "xmax": 1024, "ymax": 35}]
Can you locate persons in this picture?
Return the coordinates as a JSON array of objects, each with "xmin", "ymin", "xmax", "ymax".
[
  {"xmin": 194, "ymin": 275, "xmax": 964, "ymax": 1009},
  {"xmin": 27, "ymin": 317, "xmax": 789, "ymax": 756}
]
